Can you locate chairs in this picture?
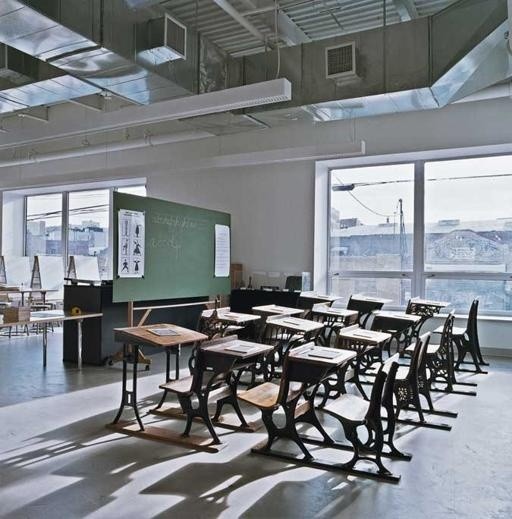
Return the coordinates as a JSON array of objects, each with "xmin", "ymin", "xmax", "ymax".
[{"xmin": 156, "ymin": 295, "xmax": 490, "ymax": 484}]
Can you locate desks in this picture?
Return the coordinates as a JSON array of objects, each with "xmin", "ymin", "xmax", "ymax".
[
  {"xmin": 110, "ymin": 322, "xmax": 209, "ymax": 438},
  {"xmin": 0, "ymin": 307, "xmax": 105, "ymax": 372},
  {"xmin": 0, "ymin": 283, "xmax": 61, "ymax": 336}
]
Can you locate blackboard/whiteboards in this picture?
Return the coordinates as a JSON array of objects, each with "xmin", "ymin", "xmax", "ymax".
[{"xmin": 111, "ymin": 191, "xmax": 231, "ymax": 304}]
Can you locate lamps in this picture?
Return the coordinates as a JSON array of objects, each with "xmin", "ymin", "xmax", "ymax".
[
  {"xmin": 2, "ymin": 0, "xmax": 294, "ymax": 153},
  {"xmin": 213, "ymin": 117, "xmax": 367, "ymax": 170}
]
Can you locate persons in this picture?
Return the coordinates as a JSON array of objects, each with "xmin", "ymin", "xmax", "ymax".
[
  {"xmin": 121, "ymin": 258, "xmax": 129, "ymax": 273},
  {"xmin": 133, "ymin": 240, "xmax": 142, "ymax": 256},
  {"xmin": 135, "ymin": 225, "xmax": 140, "ymax": 237},
  {"xmin": 132, "ymin": 259, "xmax": 141, "ymax": 274}
]
